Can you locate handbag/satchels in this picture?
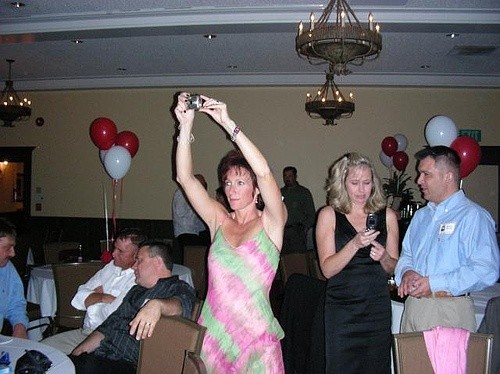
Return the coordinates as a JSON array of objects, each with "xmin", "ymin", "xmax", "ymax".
[
  {"xmin": 15, "ymin": 348, "xmax": 52, "ymax": 374},
  {"xmin": 25, "ymin": 323, "xmax": 80, "ymax": 341}
]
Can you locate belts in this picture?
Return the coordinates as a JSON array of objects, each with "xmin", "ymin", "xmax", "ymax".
[
  {"xmin": 455, "ymin": 292, "xmax": 470, "ymax": 296},
  {"xmin": 285, "ymin": 222, "xmax": 303, "ymax": 230}
]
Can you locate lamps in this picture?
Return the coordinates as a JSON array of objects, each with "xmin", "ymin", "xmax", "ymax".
[
  {"xmin": 295, "ymin": 0, "xmax": 383, "ymax": 77},
  {"xmin": 0, "ymin": 58, "xmax": 31, "ymax": 128},
  {"xmin": 305, "ymin": 64, "xmax": 355, "ymax": 126}
]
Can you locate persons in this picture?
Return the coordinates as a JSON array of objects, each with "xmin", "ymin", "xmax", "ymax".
[
  {"xmin": 67, "ymin": 239, "xmax": 196, "ymax": 374},
  {"xmin": 0, "ymin": 217, "xmax": 29, "ymax": 338},
  {"xmin": 314, "ymin": 152, "xmax": 399, "ymax": 374},
  {"xmin": 175, "ymin": 92, "xmax": 288, "ymax": 374},
  {"xmin": 172, "ymin": 174, "xmax": 233, "ymax": 266},
  {"xmin": 279, "ymin": 166, "xmax": 316, "ymax": 254},
  {"xmin": 39, "ymin": 227, "xmax": 148, "ymax": 356},
  {"xmin": 393, "ymin": 146, "xmax": 500, "ymax": 374}
]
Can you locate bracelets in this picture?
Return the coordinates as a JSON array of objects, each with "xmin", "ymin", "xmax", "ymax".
[
  {"xmin": 177, "ymin": 133, "xmax": 195, "ymax": 144},
  {"xmin": 178, "ymin": 121, "xmax": 183, "ymax": 130},
  {"xmin": 231, "ymin": 126, "xmax": 241, "ymax": 141}
]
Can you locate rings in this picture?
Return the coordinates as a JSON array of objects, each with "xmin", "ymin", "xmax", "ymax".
[
  {"xmin": 373, "ymin": 252, "xmax": 375, "ymax": 255},
  {"xmin": 145, "ymin": 323, "xmax": 150, "ymax": 325}
]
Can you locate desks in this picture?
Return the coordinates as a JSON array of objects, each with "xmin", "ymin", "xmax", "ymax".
[
  {"xmin": 0, "ymin": 334, "xmax": 76, "ymax": 374},
  {"xmin": 27, "ymin": 261, "xmax": 194, "ymax": 319},
  {"xmin": 389, "ymin": 282, "xmax": 499, "ymax": 335}
]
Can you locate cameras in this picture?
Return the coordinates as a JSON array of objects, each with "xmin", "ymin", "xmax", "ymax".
[{"xmin": 184, "ymin": 94, "xmax": 203, "ymax": 110}]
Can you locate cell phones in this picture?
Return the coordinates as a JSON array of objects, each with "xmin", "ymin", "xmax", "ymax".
[{"xmin": 365, "ymin": 213, "xmax": 378, "ymax": 234}]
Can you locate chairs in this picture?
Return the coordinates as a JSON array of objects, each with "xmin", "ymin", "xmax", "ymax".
[
  {"xmin": 52, "ymin": 263, "xmax": 105, "ymax": 334},
  {"xmin": 183, "ymin": 245, "xmax": 211, "ymax": 302},
  {"xmin": 42, "ymin": 241, "xmax": 82, "ymax": 265},
  {"xmin": 277, "ymin": 272, "xmax": 326, "ymax": 374},
  {"xmin": 136, "ymin": 311, "xmax": 209, "ymax": 374},
  {"xmin": 394, "ymin": 329, "xmax": 492, "ymax": 374},
  {"xmin": 276, "ymin": 254, "xmax": 310, "ymax": 285}
]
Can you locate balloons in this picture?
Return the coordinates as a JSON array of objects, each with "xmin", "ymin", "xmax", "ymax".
[
  {"xmin": 394, "ymin": 134, "xmax": 408, "ymax": 152},
  {"xmin": 393, "ymin": 151, "xmax": 409, "ymax": 171},
  {"xmin": 115, "ymin": 131, "xmax": 139, "ymax": 159},
  {"xmin": 425, "ymin": 115, "xmax": 457, "ymax": 148},
  {"xmin": 381, "ymin": 136, "xmax": 398, "ymax": 158},
  {"xmin": 99, "ymin": 143, "xmax": 115, "ymax": 164},
  {"xmin": 104, "ymin": 145, "xmax": 132, "ymax": 182},
  {"xmin": 450, "ymin": 135, "xmax": 481, "ymax": 179},
  {"xmin": 379, "ymin": 151, "xmax": 393, "ymax": 168},
  {"xmin": 89, "ymin": 117, "xmax": 118, "ymax": 150}
]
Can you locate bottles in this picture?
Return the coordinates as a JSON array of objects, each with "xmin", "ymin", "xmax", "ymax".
[{"xmin": 78, "ymin": 245, "xmax": 82, "ymax": 263}]
